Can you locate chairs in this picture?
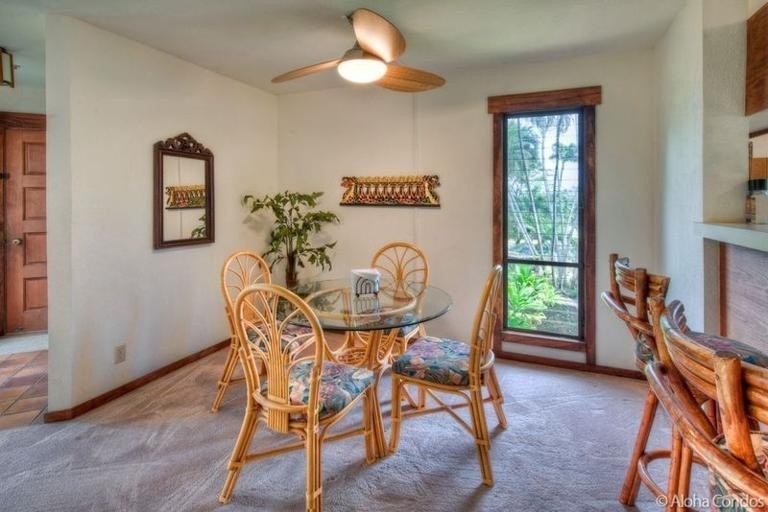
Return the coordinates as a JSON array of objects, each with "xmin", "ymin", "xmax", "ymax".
[{"xmin": 209, "ymin": 241, "xmax": 509, "ymax": 512}]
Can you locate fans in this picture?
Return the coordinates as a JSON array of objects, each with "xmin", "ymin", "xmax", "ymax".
[{"xmin": 271, "ymin": 8, "xmax": 446, "ymax": 93}]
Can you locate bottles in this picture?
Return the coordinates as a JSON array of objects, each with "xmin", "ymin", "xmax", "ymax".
[{"xmin": 746, "ymin": 179, "xmax": 768, "ymax": 225}]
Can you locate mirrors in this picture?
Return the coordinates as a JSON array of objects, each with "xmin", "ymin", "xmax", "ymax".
[{"xmin": 155, "ymin": 133, "xmax": 215, "ymax": 248}]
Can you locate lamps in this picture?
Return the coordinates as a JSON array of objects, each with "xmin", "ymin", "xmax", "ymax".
[{"xmin": 336, "ymin": 49, "xmax": 388, "ymax": 85}]
